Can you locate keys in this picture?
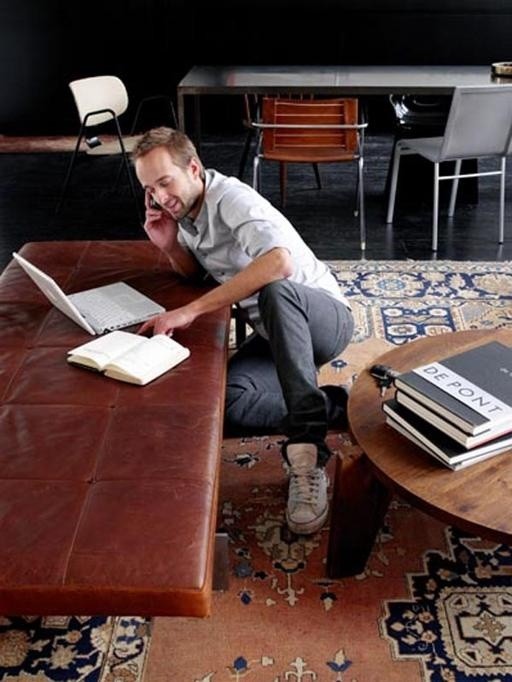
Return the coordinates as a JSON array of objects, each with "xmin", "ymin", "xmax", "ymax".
[{"xmin": 368, "ymin": 361, "xmax": 403, "ymax": 400}]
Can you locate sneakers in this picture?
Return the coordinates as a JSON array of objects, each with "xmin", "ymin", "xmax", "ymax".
[
  {"xmin": 339, "ymin": 372, "xmax": 359, "ymax": 394},
  {"xmin": 286, "ymin": 442, "xmax": 330, "ymax": 535}
]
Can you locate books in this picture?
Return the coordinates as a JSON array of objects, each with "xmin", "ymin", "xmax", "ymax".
[
  {"xmin": 383, "ymin": 339, "xmax": 512, "ymax": 475},
  {"xmin": 65, "ymin": 328, "xmax": 192, "ymax": 387}
]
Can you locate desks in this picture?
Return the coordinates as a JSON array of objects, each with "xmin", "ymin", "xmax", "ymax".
[{"xmin": 177, "ymin": 63, "xmax": 510, "ymax": 159}]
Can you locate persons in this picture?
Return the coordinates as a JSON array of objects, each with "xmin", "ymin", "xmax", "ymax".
[{"xmin": 126, "ymin": 127, "xmax": 357, "ymax": 536}]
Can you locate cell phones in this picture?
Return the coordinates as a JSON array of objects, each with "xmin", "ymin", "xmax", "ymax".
[{"xmin": 148, "ymin": 194, "xmax": 164, "ymax": 211}]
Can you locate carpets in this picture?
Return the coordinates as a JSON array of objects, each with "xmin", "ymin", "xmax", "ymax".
[{"xmin": 1, "ymin": 259, "xmax": 512, "ymax": 682}]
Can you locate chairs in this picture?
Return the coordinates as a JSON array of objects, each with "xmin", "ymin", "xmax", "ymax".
[{"xmin": 55, "ymin": 75, "xmax": 178, "ymax": 223}]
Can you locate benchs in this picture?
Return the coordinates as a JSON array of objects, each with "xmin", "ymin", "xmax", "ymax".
[{"xmin": 1, "ymin": 240, "xmax": 230, "ymax": 621}]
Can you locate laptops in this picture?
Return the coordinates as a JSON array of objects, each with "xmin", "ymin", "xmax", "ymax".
[{"xmin": 11, "ymin": 252, "xmax": 166, "ymax": 335}]
